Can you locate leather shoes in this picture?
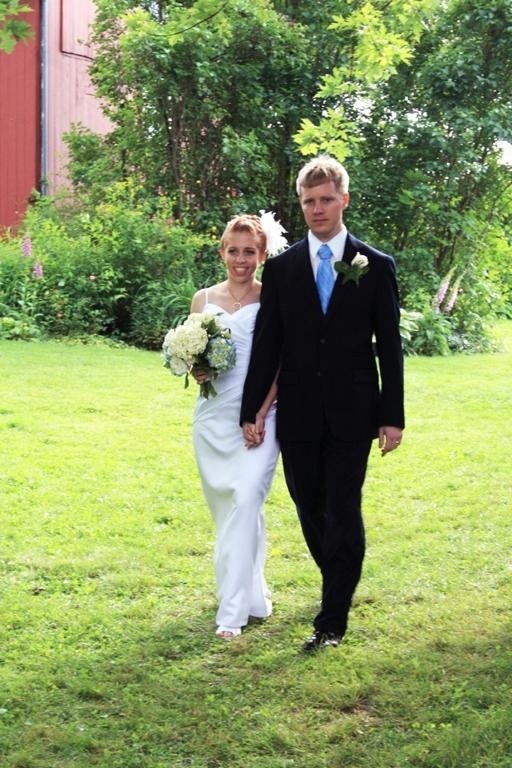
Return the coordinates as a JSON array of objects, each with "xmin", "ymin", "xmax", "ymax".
[{"xmin": 303, "ymin": 630, "xmax": 344, "ymax": 655}]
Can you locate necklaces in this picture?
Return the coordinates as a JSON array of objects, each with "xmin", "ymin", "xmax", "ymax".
[{"xmin": 227, "ymin": 287, "xmax": 253, "ymax": 312}]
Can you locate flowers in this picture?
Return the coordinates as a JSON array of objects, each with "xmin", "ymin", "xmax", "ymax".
[
  {"xmin": 333, "ymin": 252, "xmax": 371, "ymax": 285},
  {"xmin": 159, "ymin": 312, "xmax": 238, "ymax": 400}
]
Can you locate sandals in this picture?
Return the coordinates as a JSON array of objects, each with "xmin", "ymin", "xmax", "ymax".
[{"xmin": 213, "ymin": 624, "xmax": 243, "ymax": 642}]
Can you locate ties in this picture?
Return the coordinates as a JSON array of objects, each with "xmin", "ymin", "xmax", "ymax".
[{"xmin": 316, "ymin": 246, "xmax": 336, "ymax": 316}]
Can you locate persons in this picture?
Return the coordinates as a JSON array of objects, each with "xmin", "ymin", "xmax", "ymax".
[
  {"xmin": 190, "ymin": 215, "xmax": 280, "ymax": 639},
  {"xmin": 240, "ymin": 157, "xmax": 404, "ymax": 652}
]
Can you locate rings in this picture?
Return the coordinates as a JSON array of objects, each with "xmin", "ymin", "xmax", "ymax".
[{"xmin": 396, "ymin": 440, "xmax": 401, "ymax": 449}]
